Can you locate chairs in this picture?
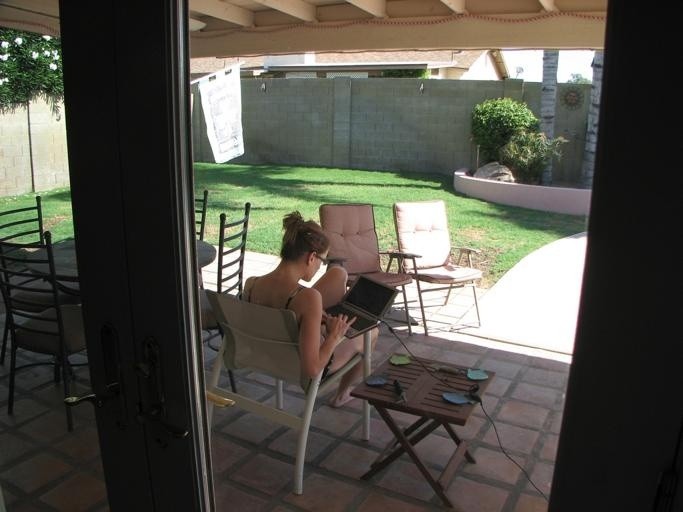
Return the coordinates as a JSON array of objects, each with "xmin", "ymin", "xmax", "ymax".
[
  {"xmin": 387, "ymin": 201, "xmax": 484, "ymax": 335},
  {"xmin": 203, "ymin": 289, "xmax": 370, "ymax": 495},
  {"xmin": 319, "ymin": 203, "xmax": 413, "ymax": 335}
]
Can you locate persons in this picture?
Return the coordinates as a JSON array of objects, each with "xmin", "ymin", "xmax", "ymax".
[{"xmin": 243, "ymin": 209, "xmax": 379, "ymax": 408}]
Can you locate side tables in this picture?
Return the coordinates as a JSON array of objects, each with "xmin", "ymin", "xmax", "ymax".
[{"xmin": 349, "ymin": 352, "xmax": 496, "ymax": 508}]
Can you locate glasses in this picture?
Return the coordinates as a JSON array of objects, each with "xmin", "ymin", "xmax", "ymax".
[{"xmin": 315, "ymin": 255, "xmax": 329, "ymax": 266}]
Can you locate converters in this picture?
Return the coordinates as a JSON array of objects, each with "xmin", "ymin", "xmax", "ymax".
[{"xmin": 469, "ymin": 384, "xmax": 482, "ymax": 402}]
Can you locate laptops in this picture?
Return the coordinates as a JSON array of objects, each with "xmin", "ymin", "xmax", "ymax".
[{"xmin": 325, "ymin": 275, "xmax": 400, "ymax": 339}]
